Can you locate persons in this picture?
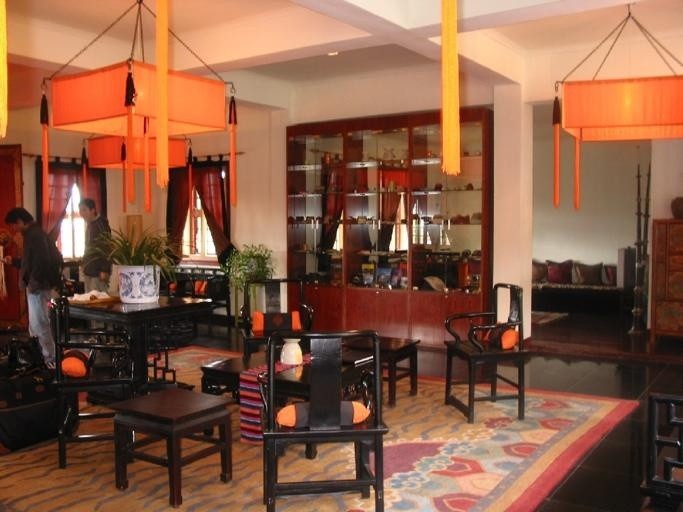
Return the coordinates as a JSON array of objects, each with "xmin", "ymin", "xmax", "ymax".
[
  {"xmin": 4, "ymin": 207, "xmax": 64, "ymax": 384},
  {"xmin": 79, "ymin": 199, "xmax": 111, "ymax": 293}
]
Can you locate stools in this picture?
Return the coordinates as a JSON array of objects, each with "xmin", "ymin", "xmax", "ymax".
[
  {"xmin": 111, "ymin": 387, "xmax": 233, "ymax": 508},
  {"xmin": 339, "ymin": 329, "xmax": 421, "ymax": 407}
]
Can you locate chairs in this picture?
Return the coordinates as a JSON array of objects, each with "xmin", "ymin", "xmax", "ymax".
[
  {"xmin": 260, "ymin": 329, "xmax": 390, "ymax": 512},
  {"xmin": 59, "ymin": 261, "xmax": 93, "ymax": 341},
  {"xmin": 238, "ymin": 276, "xmax": 314, "ymax": 355},
  {"xmin": 0, "ymin": 326, "xmax": 80, "ymax": 456},
  {"xmin": 443, "ymin": 283, "xmax": 528, "ymax": 424},
  {"xmin": 51, "ymin": 297, "xmax": 136, "ymax": 469}
]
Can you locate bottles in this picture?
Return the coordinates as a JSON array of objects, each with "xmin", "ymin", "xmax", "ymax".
[{"xmin": 321, "ymin": 152, "xmax": 340, "ymax": 165}]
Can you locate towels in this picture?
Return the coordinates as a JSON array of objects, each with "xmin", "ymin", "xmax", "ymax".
[{"xmin": 239, "ymin": 354, "xmax": 310, "ymax": 447}]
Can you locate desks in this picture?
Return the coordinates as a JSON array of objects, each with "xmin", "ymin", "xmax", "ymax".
[
  {"xmin": 202, "ymin": 353, "xmax": 355, "ymax": 458},
  {"xmin": 54, "ymin": 294, "xmax": 212, "ymax": 406}
]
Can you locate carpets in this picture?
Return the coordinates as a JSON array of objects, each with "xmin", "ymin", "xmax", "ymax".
[
  {"xmin": 530, "ymin": 310, "xmax": 570, "ymax": 326},
  {"xmin": 1, "ymin": 343, "xmax": 641, "ymax": 512}
]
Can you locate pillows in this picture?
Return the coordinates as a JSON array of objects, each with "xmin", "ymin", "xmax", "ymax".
[{"xmin": 532, "ymin": 258, "xmax": 617, "ymax": 286}]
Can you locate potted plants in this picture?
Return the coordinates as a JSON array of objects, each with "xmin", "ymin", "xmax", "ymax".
[
  {"xmin": 80, "ymin": 225, "xmax": 196, "ymax": 304},
  {"xmin": 223, "ymin": 244, "xmax": 275, "ymax": 287}
]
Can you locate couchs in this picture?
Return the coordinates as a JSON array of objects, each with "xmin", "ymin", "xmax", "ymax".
[
  {"xmin": 535, "ymin": 246, "xmax": 636, "ymax": 314},
  {"xmin": 162, "ymin": 268, "xmax": 232, "ymax": 334}
]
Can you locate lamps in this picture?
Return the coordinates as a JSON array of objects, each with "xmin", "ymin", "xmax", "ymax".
[
  {"xmin": 81, "ymin": 133, "xmax": 195, "ymax": 212},
  {"xmin": 40, "ymin": 0, "xmax": 239, "ymax": 208},
  {"xmin": 554, "ymin": 5, "xmax": 683, "ymax": 210}
]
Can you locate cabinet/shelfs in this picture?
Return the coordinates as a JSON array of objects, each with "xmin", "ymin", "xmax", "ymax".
[
  {"xmin": 646, "ymin": 218, "xmax": 683, "ymax": 353},
  {"xmin": 287, "ymin": 105, "xmax": 494, "ymax": 354}
]
